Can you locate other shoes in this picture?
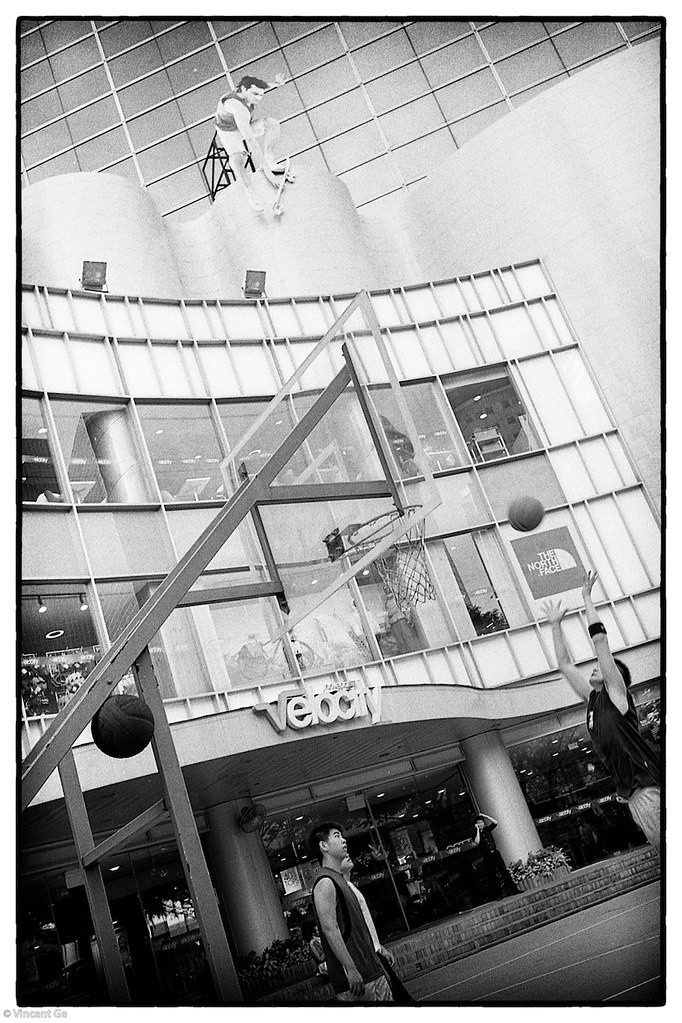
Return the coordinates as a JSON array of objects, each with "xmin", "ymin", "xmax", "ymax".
[
  {"xmin": 497, "ymin": 896, "xmax": 503, "ymax": 901},
  {"xmin": 512, "ymin": 891, "xmax": 525, "ymax": 895}
]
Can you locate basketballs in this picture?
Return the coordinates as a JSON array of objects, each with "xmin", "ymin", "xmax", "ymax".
[
  {"xmin": 507, "ymin": 496, "xmax": 544, "ymax": 532},
  {"xmin": 90, "ymin": 693, "xmax": 155, "ymax": 759}
]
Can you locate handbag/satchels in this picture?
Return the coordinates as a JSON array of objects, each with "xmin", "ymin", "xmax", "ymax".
[{"xmin": 335, "ymin": 969, "xmax": 395, "ymax": 1002}]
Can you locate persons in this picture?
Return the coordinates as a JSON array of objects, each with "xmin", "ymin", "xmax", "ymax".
[
  {"xmin": 211, "ymin": 75, "xmax": 287, "ymax": 215},
  {"xmin": 577, "ymin": 816, "xmax": 606, "ymax": 865},
  {"xmin": 348, "ymin": 583, "xmax": 422, "ymax": 662},
  {"xmin": 540, "ymin": 570, "xmax": 661, "ymax": 855},
  {"xmin": 472, "ymin": 813, "xmax": 526, "ymax": 901},
  {"xmin": 309, "ymin": 822, "xmax": 418, "ymax": 1003}
]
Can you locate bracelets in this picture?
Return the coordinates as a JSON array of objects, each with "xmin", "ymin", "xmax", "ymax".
[{"xmin": 588, "ymin": 622, "xmax": 607, "ymax": 638}]
